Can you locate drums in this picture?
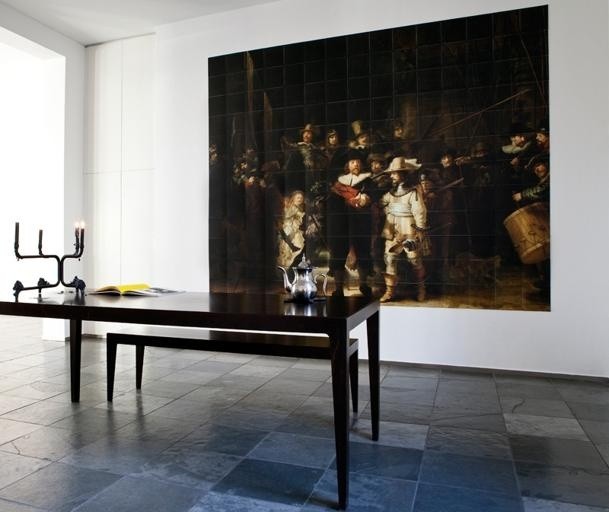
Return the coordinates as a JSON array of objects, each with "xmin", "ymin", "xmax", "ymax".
[{"xmin": 503, "ymin": 203, "xmax": 550, "ymax": 263}]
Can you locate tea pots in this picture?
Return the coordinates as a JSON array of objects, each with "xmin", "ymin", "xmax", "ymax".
[{"xmin": 276, "ymin": 251, "xmax": 330, "ymax": 306}]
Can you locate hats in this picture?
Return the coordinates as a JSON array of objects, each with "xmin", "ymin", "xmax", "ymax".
[
  {"xmin": 383, "ymin": 156, "xmax": 423, "ymax": 173},
  {"xmin": 351, "ymin": 119, "xmax": 369, "ymax": 139},
  {"xmin": 500, "ymin": 123, "xmax": 534, "ymax": 140},
  {"xmin": 300, "ymin": 123, "xmax": 317, "ymax": 135},
  {"xmin": 344, "ymin": 148, "xmax": 364, "ymax": 162},
  {"xmin": 473, "ymin": 142, "xmax": 489, "ymax": 154},
  {"xmin": 524, "ymin": 153, "xmax": 549, "ymax": 170}
]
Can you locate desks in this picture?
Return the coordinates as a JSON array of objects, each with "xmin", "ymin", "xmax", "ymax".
[{"xmin": 1, "ymin": 287, "xmax": 382, "ymax": 510}]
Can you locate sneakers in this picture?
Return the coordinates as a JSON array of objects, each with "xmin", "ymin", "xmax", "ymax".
[{"xmin": 532, "ymin": 280, "xmax": 550, "ymax": 292}]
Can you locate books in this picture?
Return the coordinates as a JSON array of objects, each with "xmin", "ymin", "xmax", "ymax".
[{"xmin": 85, "ymin": 283, "xmax": 185, "ymax": 298}]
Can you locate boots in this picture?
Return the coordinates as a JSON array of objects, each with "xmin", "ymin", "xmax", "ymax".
[
  {"xmin": 379, "ymin": 271, "xmax": 401, "ymax": 303},
  {"xmin": 357, "ymin": 269, "xmax": 372, "ymax": 295},
  {"xmin": 332, "ymin": 269, "xmax": 345, "ymax": 297},
  {"xmin": 413, "ymin": 266, "xmax": 428, "ymax": 302}
]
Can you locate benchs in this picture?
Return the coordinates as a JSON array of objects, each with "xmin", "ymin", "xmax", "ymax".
[{"xmin": 106, "ymin": 326, "xmax": 359, "ymax": 414}]
